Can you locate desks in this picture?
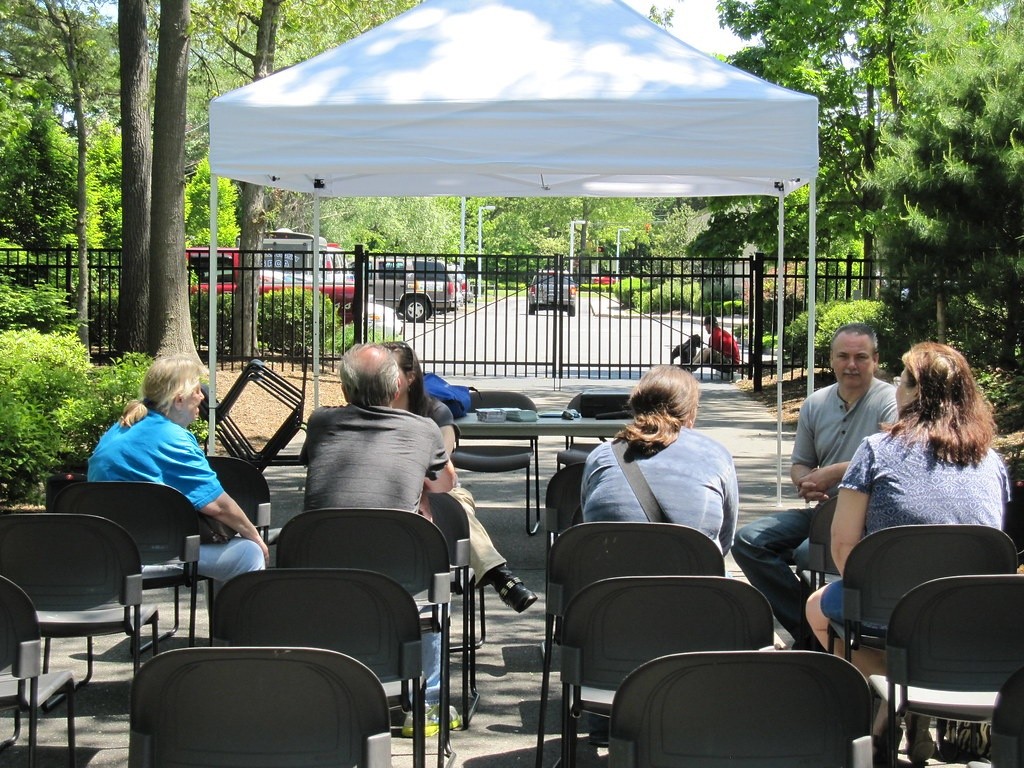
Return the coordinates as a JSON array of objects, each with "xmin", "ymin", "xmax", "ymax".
[{"xmin": 450, "ymin": 408, "xmax": 636, "ymax": 438}]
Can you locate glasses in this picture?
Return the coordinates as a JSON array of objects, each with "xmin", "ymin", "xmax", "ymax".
[
  {"xmin": 382, "ymin": 341, "xmax": 412, "ymax": 356},
  {"xmin": 893, "ymin": 376, "xmax": 914, "ymax": 388}
]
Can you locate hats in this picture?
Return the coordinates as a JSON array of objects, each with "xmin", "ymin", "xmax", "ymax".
[{"xmin": 703, "ymin": 316, "xmax": 717, "ymax": 325}]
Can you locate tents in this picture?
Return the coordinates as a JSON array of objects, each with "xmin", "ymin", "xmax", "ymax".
[{"xmin": 208, "ymin": 0, "xmax": 819, "ymax": 506}]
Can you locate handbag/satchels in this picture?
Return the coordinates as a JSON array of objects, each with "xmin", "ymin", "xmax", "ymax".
[{"xmin": 194, "ymin": 508, "xmax": 239, "ymax": 544}]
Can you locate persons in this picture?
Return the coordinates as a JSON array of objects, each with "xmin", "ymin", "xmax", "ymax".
[
  {"xmin": 380, "ymin": 342, "xmax": 538, "ymax": 613},
  {"xmin": 686, "ymin": 315, "xmax": 740, "ymax": 373},
  {"xmin": 299, "ymin": 343, "xmax": 461, "ymax": 736},
  {"xmin": 731, "ymin": 322, "xmax": 899, "ymax": 652},
  {"xmin": 87, "ymin": 355, "xmax": 269, "ymax": 647},
  {"xmin": 806, "ymin": 342, "xmax": 1011, "ymax": 763},
  {"xmin": 581, "ymin": 365, "xmax": 740, "ymax": 558}
]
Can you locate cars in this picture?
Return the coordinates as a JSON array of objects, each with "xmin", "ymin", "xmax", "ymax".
[
  {"xmin": 185, "ymin": 228, "xmax": 475, "ymax": 340},
  {"xmin": 594, "ymin": 275, "xmax": 620, "ymax": 285}
]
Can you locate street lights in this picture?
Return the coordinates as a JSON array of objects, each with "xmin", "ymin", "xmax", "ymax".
[
  {"xmin": 569, "ymin": 220, "xmax": 587, "ymax": 280},
  {"xmin": 478, "ymin": 205, "xmax": 496, "ymax": 299},
  {"xmin": 616, "ymin": 227, "xmax": 631, "ymax": 284}
]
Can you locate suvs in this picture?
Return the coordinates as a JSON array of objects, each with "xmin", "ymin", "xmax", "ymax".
[{"xmin": 529, "ymin": 269, "xmax": 577, "ymax": 317}]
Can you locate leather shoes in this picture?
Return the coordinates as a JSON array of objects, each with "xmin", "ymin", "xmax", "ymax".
[{"xmin": 488, "ymin": 565, "xmax": 539, "ymax": 613}]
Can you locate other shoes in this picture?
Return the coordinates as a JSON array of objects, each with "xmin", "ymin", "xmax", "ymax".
[{"xmin": 903, "ymin": 711, "xmax": 935, "ymax": 764}]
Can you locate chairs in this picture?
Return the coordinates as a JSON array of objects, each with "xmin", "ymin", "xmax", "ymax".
[{"xmin": 0, "ymin": 360, "xmax": 1024, "ymax": 768}]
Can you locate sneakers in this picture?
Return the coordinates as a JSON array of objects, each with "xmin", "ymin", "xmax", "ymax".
[{"xmin": 402, "ymin": 706, "xmax": 460, "ymax": 737}]
